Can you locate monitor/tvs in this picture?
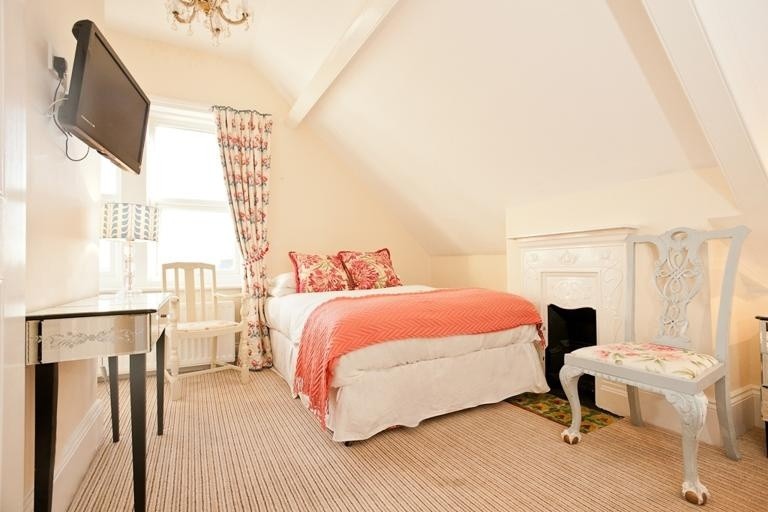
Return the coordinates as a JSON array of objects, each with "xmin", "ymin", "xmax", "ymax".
[{"xmin": 57, "ymin": 20, "xmax": 151, "ymax": 175}]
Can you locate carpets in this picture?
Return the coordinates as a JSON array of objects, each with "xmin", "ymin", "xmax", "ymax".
[{"xmin": 503, "ymin": 392, "xmax": 621, "ymax": 434}]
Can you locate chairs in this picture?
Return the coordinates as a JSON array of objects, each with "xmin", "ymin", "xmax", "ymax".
[
  {"xmin": 162, "ymin": 263, "xmax": 250, "ymax": 401},
  {"xmin": 559, "ymin": 224, "xmax": 752, "ymax": 505}
]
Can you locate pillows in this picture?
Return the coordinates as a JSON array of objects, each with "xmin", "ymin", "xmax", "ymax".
[
  {"xmin": 289, "ymin": 252, "xmax": 355, "ymax": 293},
  {"xmin": 264, "ymin": 272, "xmax": 297, "ymax": 297},
  {"xmin": 337, "ymin": 248, "xmax": 403, "ymax": 290}
]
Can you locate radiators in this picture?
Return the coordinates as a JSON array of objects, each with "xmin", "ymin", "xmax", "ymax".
[{"xmin": 105, "ymin": 301, "xmax": 235, "ymax": 380}]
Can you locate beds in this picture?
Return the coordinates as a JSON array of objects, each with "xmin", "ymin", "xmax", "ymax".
[{"xmin": 264, "ymin": 248, "xmax": 550, "ymax": 446}]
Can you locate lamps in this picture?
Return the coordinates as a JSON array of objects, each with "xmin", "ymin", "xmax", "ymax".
[
  {"xmin": 163, "ymin": 0, "xmax": 255, "ymax": 48},
  {"xmin": 99, "ymin": 202, "xmax": 159, "ymax": 297}
]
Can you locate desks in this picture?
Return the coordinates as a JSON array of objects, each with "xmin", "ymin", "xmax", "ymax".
[{"xmin": 26, "ymin": 293, "xmax": 170, "ymax": 511}]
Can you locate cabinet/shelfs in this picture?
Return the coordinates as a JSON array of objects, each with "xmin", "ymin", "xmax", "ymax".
[{"xmin": 755, "ymin": 315, "xmax": 768, "ymax": 457}]
